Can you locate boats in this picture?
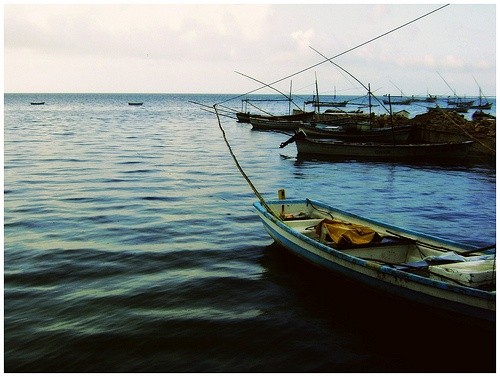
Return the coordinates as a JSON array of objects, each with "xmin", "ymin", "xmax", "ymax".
[
  {"xmin": 250, "ymin": 188, "xmax": 499, "ymax": 321},
  {"xmin": 128, "ymin": 102, "xmax": 144, "ymax": 106},
  {"xmin": 30, "ymin": 102, "xmax": 45, "ymax": 105},
  {"xmin": 188, "ymin": 46, "xmax": 496, "ymax": 176}
]
